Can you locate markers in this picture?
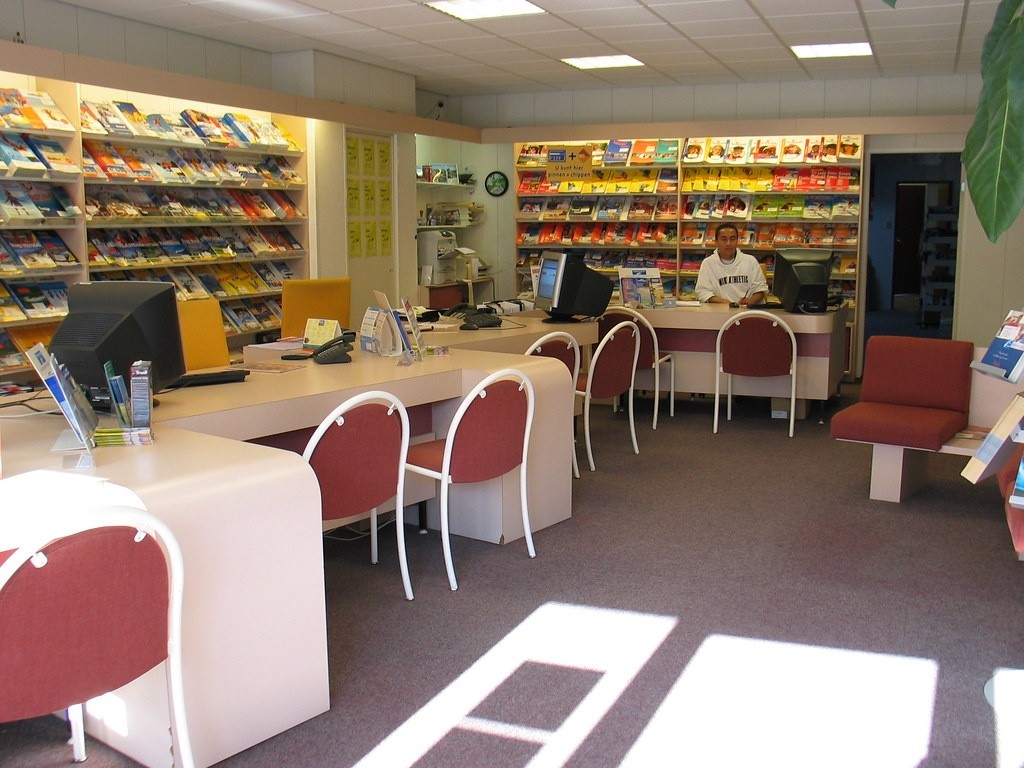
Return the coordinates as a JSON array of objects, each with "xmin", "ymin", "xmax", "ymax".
[{"xmin": 406, "ymin": 326, "xmax": 433, "ymax": 334}]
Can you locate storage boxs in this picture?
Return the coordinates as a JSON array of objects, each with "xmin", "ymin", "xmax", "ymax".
[{"xmin": 770, "ymin": 397, "xmax": 811, "ymax": 420}]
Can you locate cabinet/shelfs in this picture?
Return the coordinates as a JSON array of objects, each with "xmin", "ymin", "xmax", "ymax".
[
  {"xmin": 1, "ymin": 71, "xmax": 318, "ymax": 379},
  {"xmin": 514, "ymin": 135, "xmax": 866, "ymax": 382},
  {"xmin": 415, "ymin": 180, "xmax": 493, "ymax": 288}
]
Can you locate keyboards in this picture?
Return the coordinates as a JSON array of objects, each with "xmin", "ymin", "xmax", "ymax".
[
  {"xmin": 747, "ymin": 304, "xmax": 784, "ymax": 310},
  {"xmin": 463, "ymin": 314, "xmax": 502, "ymax": 327},
  {"xmin": 166, "ymin": 370, "xmax": 250, "ymax": 389}
]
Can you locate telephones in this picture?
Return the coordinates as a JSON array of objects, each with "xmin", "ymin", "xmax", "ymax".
[
  {"xmin": 444, "ymin": 303, "xmax": 478, "ymax": 319},
  {"xmin": 463, "ymin": 314, "xmax": 502, "ymax": 327},
  {"xmin": 314, "ymin": 334, "xmax": 355, "ymax": 363},
  {"xmin": 827, "ymin": 294, "xmax": 843, "ymax": 305}
]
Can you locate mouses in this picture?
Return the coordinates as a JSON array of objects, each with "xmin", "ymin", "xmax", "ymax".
[
  {"xmin": 729, "ymin": 302, "xmax": 739, "ymax": 308},
  {"xmin": 460, "ymin": 323, "xmax": 479, "ymax": 330}
]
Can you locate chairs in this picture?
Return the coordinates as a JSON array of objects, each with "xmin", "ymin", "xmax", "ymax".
[
  {"xmin": 522, "ymin": 330, "xmax": 582, "ymax": 479},
  {"xmin": 575, "ymin": 320, "xmax": 641, "ymax": 471},
  {"xmin": 0, "ymin": 504, "xmax": 195, "ymax": 768},
  {"xmin": 591, "ymin": 306, "xmax": 675, "ymax": 430},
  {"xmin": 712, "ymin": 310, "xmax": 798, "ymax": 439},
  {"xmin": 406, "ymin": 369, "xmax": 536, "ymax": 592},
  {"xmin": 302, "ymin": 390, "xmax": 414, "ymax": 602},
  {"xmin": 828, "ymin": 334, "xmax": 975, "ymax": 506}
]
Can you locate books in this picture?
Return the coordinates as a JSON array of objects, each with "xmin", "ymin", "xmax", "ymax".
[
  {"xmin": 0, "ymin": 277, "xmax": 69, "ymax": 370},
  {"xmin": 25, "ymin": 342, "xmax": 155, "ymax": 448},
  {"xmin": 89, "ymin": 261, "xmax": 296, "ymax": 335},
  {"xmin": 87, "ymin": 225, "xmax": 305, "ymax": 268},
  {"xmin": 961, "ymin": 392, "xmax": 1024, "ymax": 485},
  {"xmin": 0, "ymin": 229, "xmax": 83, "ymax": 276},
  {"xmin": 0, "ymin": 88, "xmax": 75, "ymax": 134},
  {"xmin": 243, "ymin": 338, "xmax": 309, "ymax": 365},
  {"xmin": 0, "ymin": 132, "xmax": 82, "ymax": 178},
  {"xmin": 79, "ymin": 99, "xmax": 306, "ymax": 154},
  {"xmin": 82, "ymin": 140, "xmax": 306, "ymax": 189},
  {"xmin": 679, "ymin": 135, "xmax": 861, "ymax": 308},
  {"xmin": 84, "ymin": 184, "xmax": 306, "ymax": 224},
  {"xmin": 0, "ymin": 180, "xmax": 83, "ymax": 226},
  {"xmin": 517, "ymin": 139, "xmax": 677, "ymax": 298},
  {"xmin": 980, "ymin": 309, "xmax": 1024, "ymax": 383}
]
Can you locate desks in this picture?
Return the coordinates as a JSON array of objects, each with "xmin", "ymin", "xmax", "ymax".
[{"xmin": 0, "ymin": 310, "xmax": 600, "ymax": 768}]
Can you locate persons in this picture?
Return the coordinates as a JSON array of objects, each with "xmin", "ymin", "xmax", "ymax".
[{"xmin": 694, "ymin": 225, "xmax": 769, "ymax": 305}]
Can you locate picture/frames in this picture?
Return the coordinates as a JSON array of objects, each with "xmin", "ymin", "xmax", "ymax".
[{"xmin": 485, "ymin": 172, "xmax": 509, "ymax": 196}]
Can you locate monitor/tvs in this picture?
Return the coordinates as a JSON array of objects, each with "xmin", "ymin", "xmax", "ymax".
[
  {"xmin": 772, "ymin": 248, "xmax": 834, "ymax": 315},
  {"xmin": 49, "ymin": 280, "xmax": 186, "ymax": 415},
  {"xmin": 534, "ymin": 252, "xmax": 615, "ymax": 324}
]
